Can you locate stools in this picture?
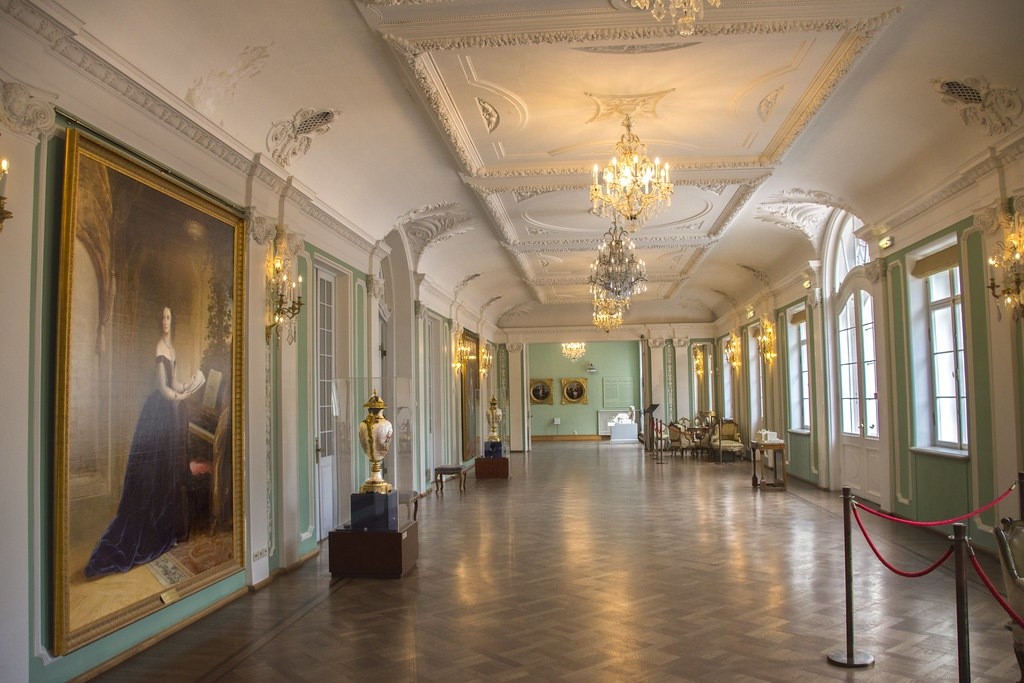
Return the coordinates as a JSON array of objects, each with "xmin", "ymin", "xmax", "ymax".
[
  {"xmin": 434, "ymin": 464, "xmax": 467, "ymax": 493},
  {"xmin": 399, "ymin": 489, "xmax": 420, "ymax": 521}
]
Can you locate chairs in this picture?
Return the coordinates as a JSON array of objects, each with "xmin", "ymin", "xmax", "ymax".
[
  {"xmin": 708, "ymin": 418, "xmax": 744, "ymax": 463},
  {"xmin": 654, "ymin": 421, "xmax": 670, "ymax": 451},
  {"xmin": 667, "ymin": 422, "xmax": 696, "ymax": 457},
  {"xmin": 674, "ymin": 420, "xmax": 692, "ymax": 442}
]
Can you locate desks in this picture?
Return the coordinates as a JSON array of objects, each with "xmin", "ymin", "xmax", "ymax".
[
  {"xmin": 686, "ymin": 427, "xmax": 709, "ymax": 458},
  {"xmin": 750, "ymin": 439, "xmax": 788, "ymax": 492}
]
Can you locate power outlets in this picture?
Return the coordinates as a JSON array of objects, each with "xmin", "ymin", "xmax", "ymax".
[
  {"xmin": 253, "ymin": 551, "xmax": 260, "ymax": 561},
  {"xmin": 260, "ymin": 548, "xmax": 268, "ymax": 558}
]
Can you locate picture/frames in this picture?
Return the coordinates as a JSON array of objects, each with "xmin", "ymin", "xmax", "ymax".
[
  {"xmin": 560, "ymin": 377, "xmax": 588, "ymax": 405},
  {"xmin": 44, "ymin": 128, "xmax": 248, "ymax": 658},
  {"xmin": 530, "ymin": 378, "xmax": 554, "ymax": 405}
]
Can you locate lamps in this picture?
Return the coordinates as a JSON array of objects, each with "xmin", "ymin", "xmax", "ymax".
[
  {"xmin": 454, "ymin": 339, "xmax": 471, "ymax": 375},
  {"xmin": 985, "ymin": 231, "xmax": 1024, "ymax": 323},
  {"xmin": 625, "ymin": 0, "xmax": 721, "ymax": 37},
  {"xmin": 591, "ymin": 312, "xmax": 624, "ymax": 335},
  {"xmin": 586, "ymin": 218, "xmax": 648, "ymax": 299},
  {"xmin": 562, "ymin": 342, "xmax": 586, "ymax": 362},
  {"xmin": 589, "ymin": 113, "xmax": 676, "ymax": 233},
  {"xmin": 0, "ymin": 157, "xmax": 13, "ymax": 231},
  {"xmin": 266, "ymin": 273, "xmax": 306, "ymax": 350},
  {"xmin": 589, "ymin": 285, "xmax": 631, "ymax": 316}
]
